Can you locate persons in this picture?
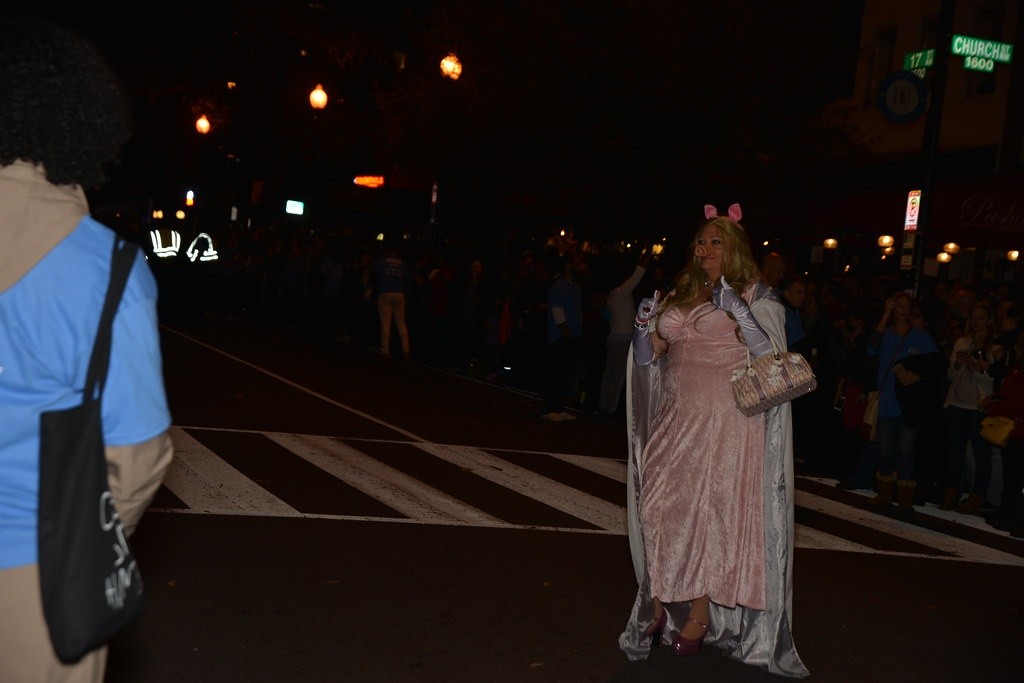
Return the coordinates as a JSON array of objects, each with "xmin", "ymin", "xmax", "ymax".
[
  {"xmin": 618, "ymin": 202, "xmax": 810, "ymax": 680},
  {"xmin": 133, "ymin": 219, "xmax": 1024, "ymax": 533},
  {"xmin": 0, "ymin": 20, "xmax": 173, "ymax": 683}
]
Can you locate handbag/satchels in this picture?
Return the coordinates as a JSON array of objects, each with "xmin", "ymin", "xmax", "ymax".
[
  {"xmin": 862, "ymin": 391, "xmax": 881, "ymax": 443},
  {"xmin": 733, "ymin": 336, "xmax": 817, "ymax": 416},
  {"xmin": 953, "ymin": 371, "xmax": 993, "ymax": 403},
  {"xmin": 980, "ymin": 414, "xmax": 1015, "ymax": 447},
  {"xmin": 36, "ymin": 235, "xmax": 144, "ymax": 665}
]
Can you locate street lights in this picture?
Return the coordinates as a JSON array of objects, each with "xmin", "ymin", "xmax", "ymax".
[
  {"xmin": 439, "ymin": 51, "xmax": 463, "ymax": 239},
  {"xmin": 191, "ymin": 112, "xmax": 213, "ymax": 240},
  {"xmin": 302, "ymin": 81, "xmax": 329, "ymax": 251}
]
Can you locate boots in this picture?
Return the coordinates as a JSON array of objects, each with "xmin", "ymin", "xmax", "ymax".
[
  {"xmin": 896, "ymin": 479, "xmax": 916, "ymax": 517},
  {"xmin": 866, "ymin": 470, "xmax": 898, "ymax": 507}
]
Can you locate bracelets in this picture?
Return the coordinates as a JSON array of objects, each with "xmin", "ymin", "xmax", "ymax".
[{"xmin": 634, "ymin": 316, "xmax": 649, "ymax": 330}]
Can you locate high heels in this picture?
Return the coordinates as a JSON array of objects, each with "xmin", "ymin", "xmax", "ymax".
[
  {"xmin": 672, "ymin": 616, "xmax": 713, "ymax": 655},
  {"xmin": 645, "ymin": 609, "xmax": 668, "ymax": 647}
]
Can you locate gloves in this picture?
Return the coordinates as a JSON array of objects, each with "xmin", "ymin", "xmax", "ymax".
[
  {"xmin": 632, "ymin": 290, "xmax": 660, "ymax": 367},
  {"xmin": 712, "ymin": 275, "xmax": 773, "ymax": 355}
]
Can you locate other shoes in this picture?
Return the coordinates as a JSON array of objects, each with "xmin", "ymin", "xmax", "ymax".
[
  {"xmin": 940, "ymin": 488, "xmax": 1024, "ymax": 538},
  {"xmin": 593, "ymin": 410, "xmax": 612, "ymax": 424},
  {"xmin": 547, "ymin": 410, "xmax": 576, "ymax": 422}
]
